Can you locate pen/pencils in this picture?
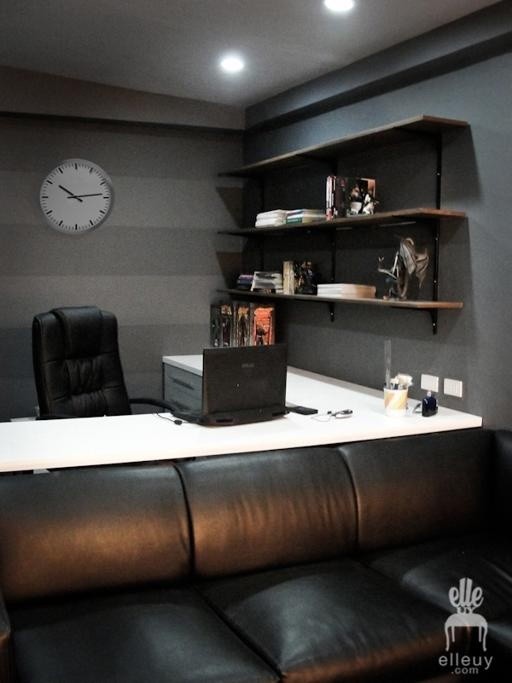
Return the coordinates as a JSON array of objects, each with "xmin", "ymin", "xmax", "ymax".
[{"xmin": 390, "ymin": 381, "xmax": 408, "ymax": 390}]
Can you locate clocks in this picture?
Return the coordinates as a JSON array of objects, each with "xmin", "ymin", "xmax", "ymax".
[{"xmin": 38, "ymin": 158, "xmax": 115, "ymax": 234}]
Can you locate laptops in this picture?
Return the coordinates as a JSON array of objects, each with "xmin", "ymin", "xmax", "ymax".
[{"xmin": 170, "ymin": 342, "xmax": 288, "ymax": 426}]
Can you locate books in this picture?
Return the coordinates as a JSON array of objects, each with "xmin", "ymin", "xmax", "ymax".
[{"xmin": 237, "ymin": 171, "xmax": 376, "ymax": 298}]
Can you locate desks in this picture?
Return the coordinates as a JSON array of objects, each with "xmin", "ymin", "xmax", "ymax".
[{"xmin": 0, "ymin": 353, "xmax": 483, "ymax": 476}]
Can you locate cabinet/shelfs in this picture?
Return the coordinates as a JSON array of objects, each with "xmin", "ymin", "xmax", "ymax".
[{"xmin": 214, "ymin": 114, "xmax": 468, "ymax": 334}]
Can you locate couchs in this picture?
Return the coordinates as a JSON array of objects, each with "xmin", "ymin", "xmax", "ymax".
[{"xmin": 0, "ymin": 429, "xmax": 511, "ymax": 683}]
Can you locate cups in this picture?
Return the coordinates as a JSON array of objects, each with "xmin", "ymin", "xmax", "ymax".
[{"xmin": 384, "ymin": 386, "xmax": 408, "ymax": 416}]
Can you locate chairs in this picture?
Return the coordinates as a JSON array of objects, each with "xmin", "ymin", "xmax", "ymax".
[{"xmin": 31, "ymin": 304, "xmax": 179, "ymax": 420}]
